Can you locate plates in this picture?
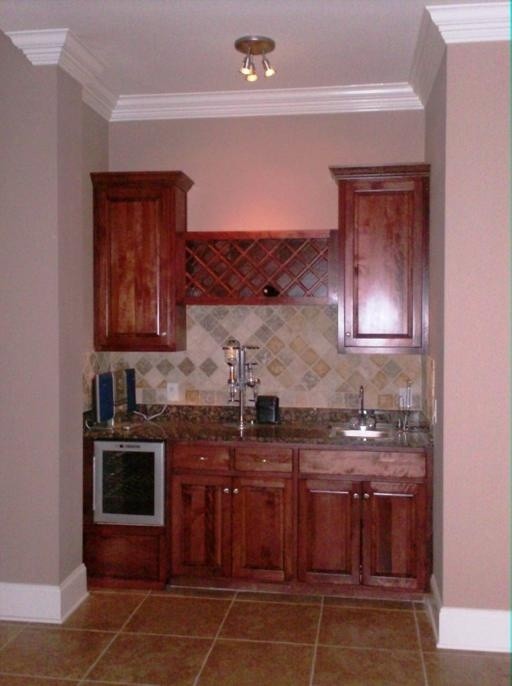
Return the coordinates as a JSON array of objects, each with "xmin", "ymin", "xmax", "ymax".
[
  {"xmin": 296, "ymin": 443, "xmax": 431, "ymax": 593},
  {"xmin": 330, "ymin": 163, "xmax": 427, "ymax": 354},
  {"xmin": 83, "ymin": 525, "xmax": 166, "ymax": 591},
  {"xmin": 91, "ymin": 171, "xmax": 194, "ymax": 353},
  {"xmin": 167, "ymin": 443, "xmax": 296, "ymax": 582}
]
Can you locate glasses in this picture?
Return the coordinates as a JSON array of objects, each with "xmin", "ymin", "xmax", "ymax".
[{"xmin": 236, "ymin": 37, "xmax": 277, "ymax": 81}]
